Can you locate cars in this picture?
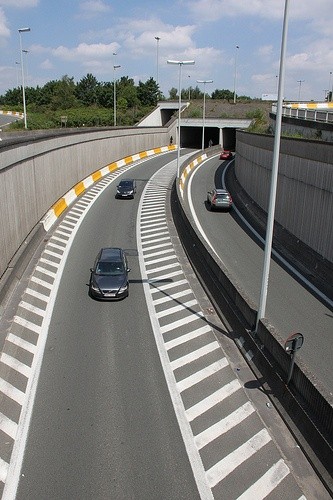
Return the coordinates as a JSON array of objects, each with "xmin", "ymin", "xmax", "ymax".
[
  {"xmin": 115, "ymin": 178, "xmax": 136, "ymax": 200},
  {"xmin": 220, "ymin": 151, "xmax": 232, "ymax": 160},
  {"xmin": 88, "ymin": 248, "xmax": 131, "ymax": 301},
  {"xmin": 207, "ymin": 188, "xmax": 233, "ymax": 212}
]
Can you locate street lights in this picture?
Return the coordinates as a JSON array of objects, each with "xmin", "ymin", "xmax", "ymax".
[
  {"xmin": 18, "ymin": 27, "xmax": 30, "ymax": 129},
  {"xmin": 297, "ymin": 80, "xmax": 304, "ymax": 101},
  {"xmin": 154, "ymin": 36, "xmax": 160, "ymax": 101},
  {"xmin": 197, "ymin": 80, "xmax": 213, "ymax": 150},
  {"xmin": 167, "ymin": 60, "xmax": 196, "ymax": 180},
  {"xmin": 233, "ymin": 46, "xmax": 239, "ymax": 104},
  {"xmin": 113, "ymin": 65, "xmax": 120, "ymax": 126}
]
